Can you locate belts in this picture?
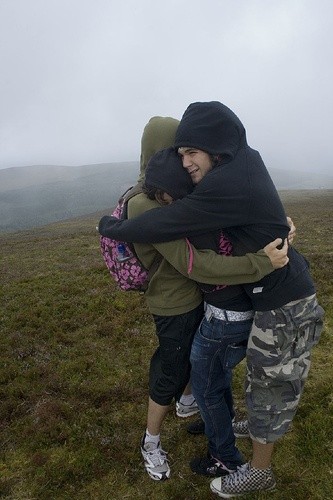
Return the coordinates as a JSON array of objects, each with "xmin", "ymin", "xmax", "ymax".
[{"xmin": 203, "ymin": 301, "xmax": 255, "ymax": 323}]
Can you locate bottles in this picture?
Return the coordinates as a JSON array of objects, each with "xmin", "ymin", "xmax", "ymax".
[{"xmin": 118, "ymin": 244, "xmax": 130, "ymax": 258}]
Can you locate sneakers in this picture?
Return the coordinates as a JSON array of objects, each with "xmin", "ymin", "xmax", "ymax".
[
  {"xmin": 140, "ymin": 429, "xmax": 170, "ymax": 481},
  {"xmin": 189, "ymin": 455, "xmax": 248, "ymax": 477},
  {"xmin": 187, "ymin": 418, "xmax": 205, "ymax": 433},
  {"xmin": 175, "ymin": 398, "xmax": 200, "ymax": 417}
]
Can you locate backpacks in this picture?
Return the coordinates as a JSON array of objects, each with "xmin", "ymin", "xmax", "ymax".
[{"xmin": 99, "ymin": 186, "xmax": 163, "ymax": 293}]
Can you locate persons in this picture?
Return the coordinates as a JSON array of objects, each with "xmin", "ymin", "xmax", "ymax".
[
  {"xmin": 126, "ymin": 115, "xmax": 295, "ymax": 482},
  {"xmin": 95, "ymin": 103, "xmax": 324, "ymax": 498},
  {"xmin": 143, "ymin": 145, "xmax": 257, "ymax": 476}
]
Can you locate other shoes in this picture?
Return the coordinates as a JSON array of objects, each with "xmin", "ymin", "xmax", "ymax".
[
  {"xmin": 210, "ymin": 460, "xmax": 277, "ymax": 498},
  {"xmin": 231, "ymin": 420, "xmax": 250, "ymax": 438}
]
